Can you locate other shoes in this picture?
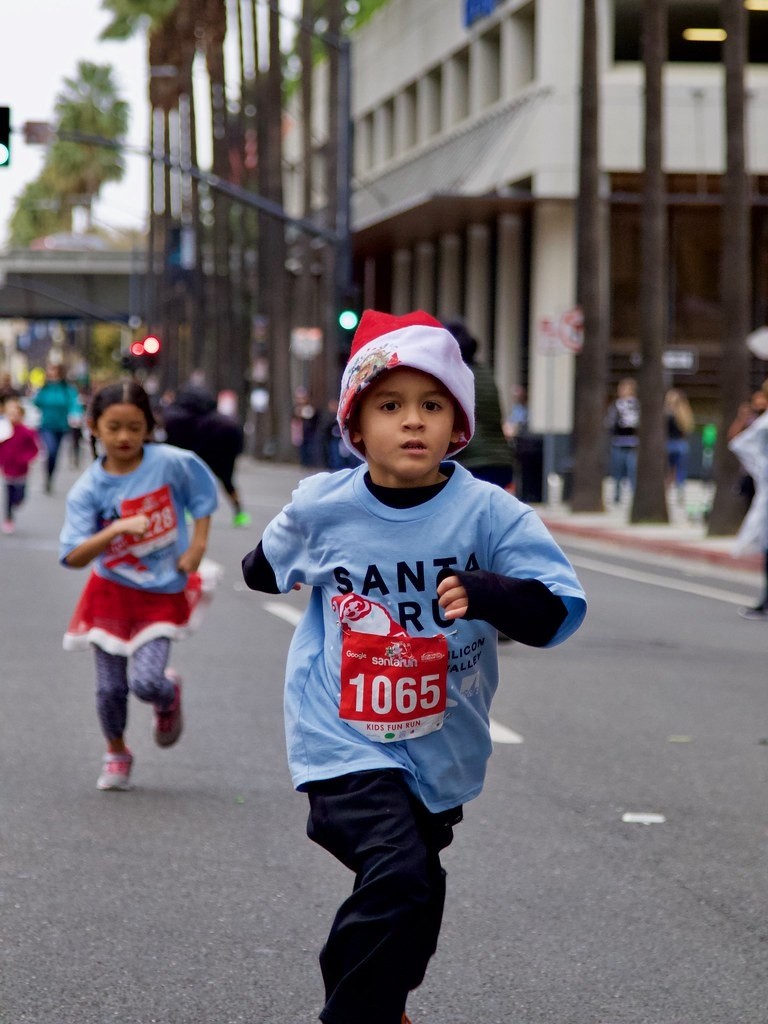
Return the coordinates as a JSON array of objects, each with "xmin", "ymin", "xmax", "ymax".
[
  {"xmin": 737, "ymin": 603, "xmax": 768, "ymax": 620},
  {"xmin": 231, "ymin": 513, "xmax": 252, "ymax": 529},
  {"xmin": 503, "ymin": 483, "xmax": 516, "ymax": 493},
  {"xmin": 3, "ymin": 518, "xmax": 17, "ymax": 535}
]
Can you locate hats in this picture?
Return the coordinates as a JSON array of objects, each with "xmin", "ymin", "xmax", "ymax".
[
  {"xmin": 509, "ymin": 384, "xmax": 527, "ymax": 395},
  {"xmin": 337, "ymin": 309, "xmax": 476, "ymax": 462}
]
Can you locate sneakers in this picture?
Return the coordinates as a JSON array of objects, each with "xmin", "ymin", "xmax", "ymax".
[
  {"xmin": 153, "ymin": 669, "xmax": 185, "ymax": 746},
  {"xmin": 96, "ymin": 747, "xmax": 134, "ymax": 791}
]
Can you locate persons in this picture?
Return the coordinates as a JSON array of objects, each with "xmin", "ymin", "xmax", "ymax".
[
  {"xmin": 242, "ymin": 310, "xmax": 588, "ymax": 1024},
  {"xmin": 0, "ymin": 351, "xmax": 366, "ymax": 534},
  {"xmin": 444, "ymin": 319, "xmax": 768, "ymax": 623},
  {"xmin": 57, "ymin": 380, "xmax": 222, "ymax": 793}
]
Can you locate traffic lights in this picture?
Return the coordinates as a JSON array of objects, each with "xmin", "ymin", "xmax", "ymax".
[{"xmin": 0, "ymin": 105, "xmax": 10, "ymax": 167}]
